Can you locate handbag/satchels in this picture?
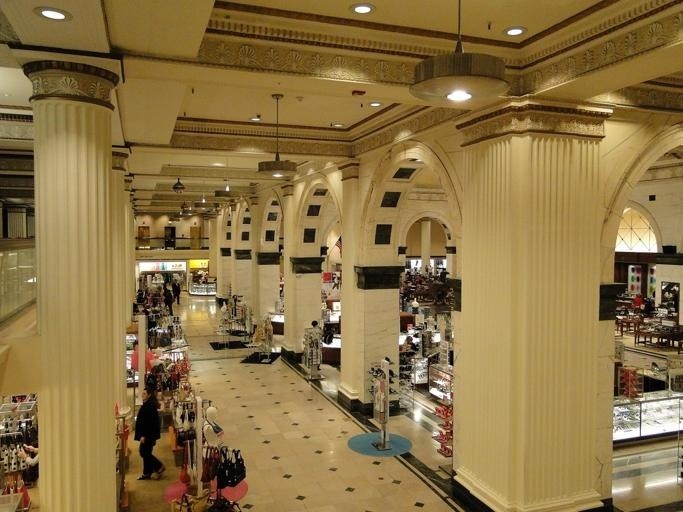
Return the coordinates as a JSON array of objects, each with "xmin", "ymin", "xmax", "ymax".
[
  {"xmin": 201, "ymin": 445, "xmax": 246, "ymax": 486},
  {"xmin": 3, "ymin": 480, "xmax": 30, "ymax": 506},
  {"xmin": 172, "ymin": 427, "xmax": 198, "ymax": 483},
  {"xmin": 171, "ymin": 488, "xmax": 242, "ymax": 512}
]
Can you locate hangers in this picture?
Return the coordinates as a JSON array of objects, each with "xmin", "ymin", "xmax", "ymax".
[{"xmin": 173, "ymin": 400, "xmax": 213, "ymax": 413}]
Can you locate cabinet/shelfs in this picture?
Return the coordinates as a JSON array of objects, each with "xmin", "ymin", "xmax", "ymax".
[
  {"xmin": 188, "ymin": 281, "xmax": 216, "ymax": 297},
  {"xmin": 429, "ymin": 364, "xmax": 453, "ymax": 402},
  {"xmin": 612, "ymin": 387, "xmax": 683, "ymax": 451}
]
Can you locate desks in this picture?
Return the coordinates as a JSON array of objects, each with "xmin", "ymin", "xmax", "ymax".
[{"xmin": 616, "ymin": 310, "xmax": 683, "ymax": 355}]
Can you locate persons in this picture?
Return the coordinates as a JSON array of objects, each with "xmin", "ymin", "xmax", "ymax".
[
  {"xmin": 633, "ymin": 293, "xmax": 642, "ymax": 314},
  {"xmin": 401, "ymin": 270, "xmax": 451, "ymax": 307},
  {"xmin": 312, "ymin": 320, "xmax": 323, "ymax": 370},
  {"xmin": 192, "ymin": 270, "xmax": 208, "ymax": 285},
  {"xmin": 402, "ymin": 337, "xmax": 419, "ymax": 366},
  {"xmin": 650, "ymin": 291, "xmax": 655, "ymax": 310},
  {"xmin": 17, "ymin": 444, "xmax": 39, "ymax": 467},
  {"xmin": 133, "ymin": 271, "xmax": 181, "ymax": 480}
]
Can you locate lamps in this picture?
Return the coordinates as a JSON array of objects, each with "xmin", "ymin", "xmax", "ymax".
[
  {"xmin": 167, "ymin": 156, "xmax": 241, "ymax": 223},
  {"xmin": 250, "ymin": 92, "xmax": 303, "ymax": 180},
  {"xmin": 400, "ymin": 1, "xmax": 512, "ymax": 107}
]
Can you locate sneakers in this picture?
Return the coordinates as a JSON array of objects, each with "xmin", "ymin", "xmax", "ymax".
[{"xmin": 137, "ymin": 474, "xmax": 150, "ymax": 480}]
